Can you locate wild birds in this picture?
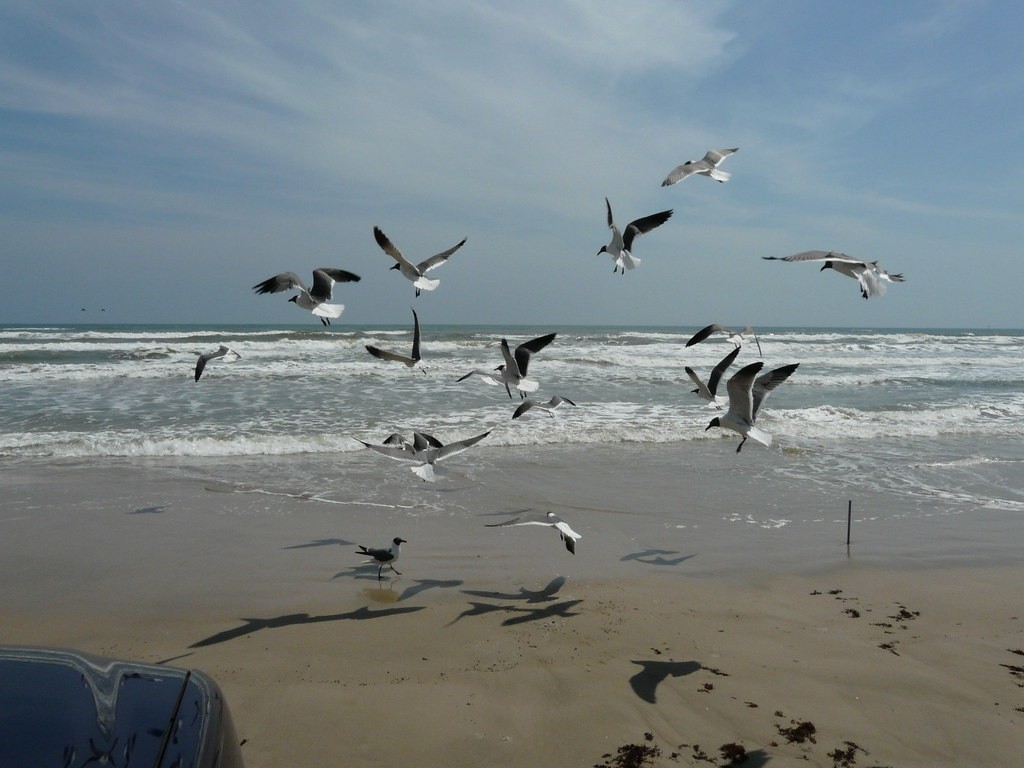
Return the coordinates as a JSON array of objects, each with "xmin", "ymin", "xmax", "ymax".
[
  {"xmin": 597, "ymin": 197, "xmax": 674, "ymax": 275},
  {"xmin": 762, "ymin": 250, "xmax": 906, "ymax": 303},
  {"xmin": 512, "ymin": 395, "xmax": 576, "ymax": 420},
  {"xmin": 484, "ymin": 512, "xmax": 581, "ymax": 555},
  {"xmin": 194, "ymin": 346, "xmax": 240, "ymax": 382},
  {"xmin": 352, "ymin": 427, "xmax": 494, "ymax": 483},
  {"xmin": 662, "ymin": 148, "xmax": 739, "ymax": 187},
  {"xmin": 685, "ymin": 345, "xmax": 742, "ymax": 410},
  {"xmin": 355, "ymin": 537, "xmax": 406, "ymax": 579},
  {"xmin": 705, "ymin": 362, "xmax": 799, "ymax": 454},
  {"xmin": 456, "ymin": 333, "xmax": 557, "ymax": 398},
  {"xmin": 365, "ymin": 308, "xmax": 429, "ymax": 374},
  {"xmin": 251, "ymin": 268, "xmax": 360, "ymax": 326},
  {"xmin": 373, "ymin": 226, "xmax": 468, "ymax": 298},
  {"xmin": 686, "ymin": 323, "xmax": 762, "ymax": 358}
]
